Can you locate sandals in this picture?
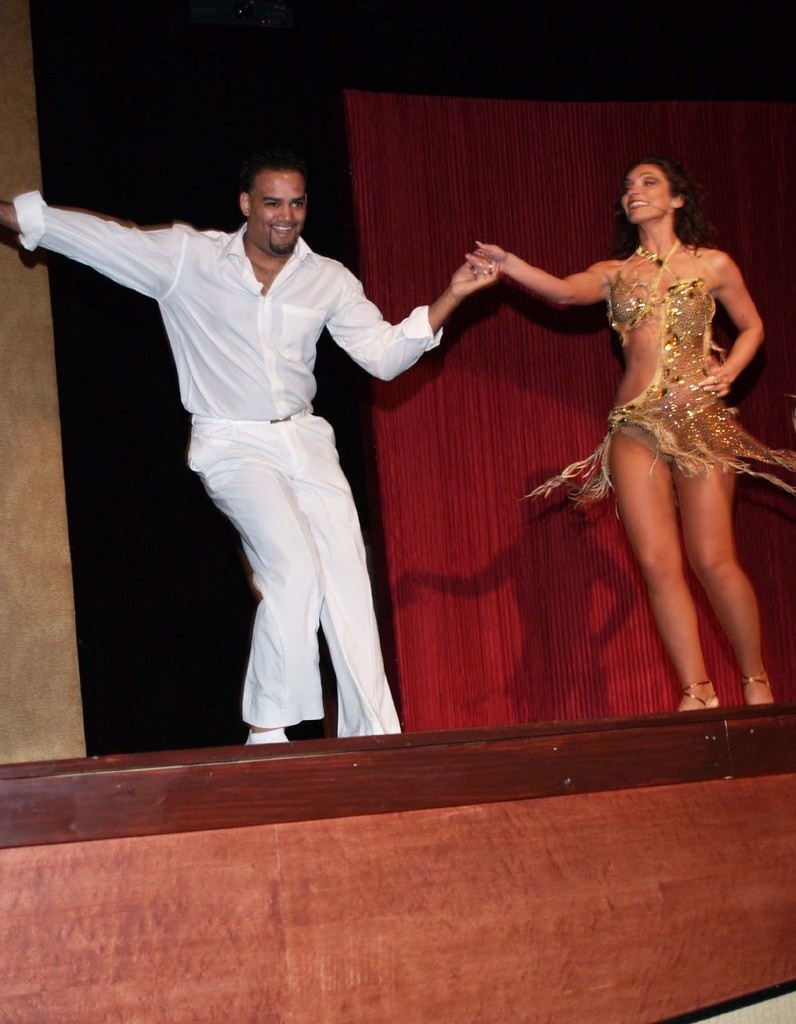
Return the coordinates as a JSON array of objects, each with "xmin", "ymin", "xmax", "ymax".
[
  {"xmin": 741, "ymin": 667, "xmax": 773, "ymax": 705},
  {"xmin": 677, "ymin": 681, "xmax": 720, "ymax": 709}
]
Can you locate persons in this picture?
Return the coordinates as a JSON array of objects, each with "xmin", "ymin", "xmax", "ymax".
[
  {"xmin": 0, "ymin": 150, "xmax": 499, "ymax": 744},
  {"xmin": 465, "ymin": 150, "xmax": 796, "ymax": 716}
]
